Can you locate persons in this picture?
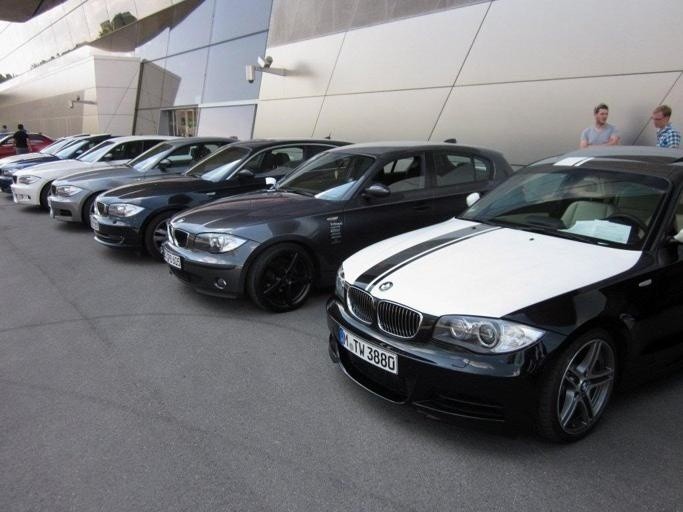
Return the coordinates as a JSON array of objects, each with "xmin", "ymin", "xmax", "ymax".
[
  {"xmin": 2, "ymin": 125, "xmax": 9, "ymax": 132},
  {"xmin": 13, "ymin": 124, "xmax": 32, "ymax": 154},
  {"xmin": 652, "ymin": 105, "xmax": 680, "ymax": 148},
  {"xmin": 580, "ymin": 102, "xmax": 621, "ymax": 149}
]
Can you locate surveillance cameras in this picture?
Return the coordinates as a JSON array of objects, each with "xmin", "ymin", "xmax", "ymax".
[
  {"xmin": 68, "ymin": 101, "xmax": 72, "ymax": 108},
  {"xmin": 246, "ymin": 65, "xmax": 254, "ymax": 84},
  {"xmin": 258, "ymin": 56, "xmax": 270, "ymax": 69},
  {"xmin": 75, "ymin": 95, "xmax": 81, "ymax": 101}
]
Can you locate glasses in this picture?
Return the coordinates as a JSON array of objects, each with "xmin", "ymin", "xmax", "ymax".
[{"xmin": 651, "ymin": 116, "xmax": 665, "ymax": 121}]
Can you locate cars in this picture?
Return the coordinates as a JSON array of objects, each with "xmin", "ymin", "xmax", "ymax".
[
  {"xmin": 90, "ymin": 141, "xmax": 338, "ymax": 263},
  {"xmin": 164, "ymin": 143, "xmax": 520, "ymax": 315},
  {"xmin": 49, "ymin": 137, "xmax": 233, "ymax": 224},
  {"xmin": 12, "ymin": 134, "xmax": 182, "ymax": 208},
  {"xmin": 328, "ymin": 143, "xmax": 682, "ymax": 441},
  {"xmin": 1, "ymin": 131, "xmax": 108, "ymax": 191}
]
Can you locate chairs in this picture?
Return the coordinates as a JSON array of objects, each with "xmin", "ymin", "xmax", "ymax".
[{"xmin": 561, "ymin": 176, "xmax": 611, "ymax": 230}]
